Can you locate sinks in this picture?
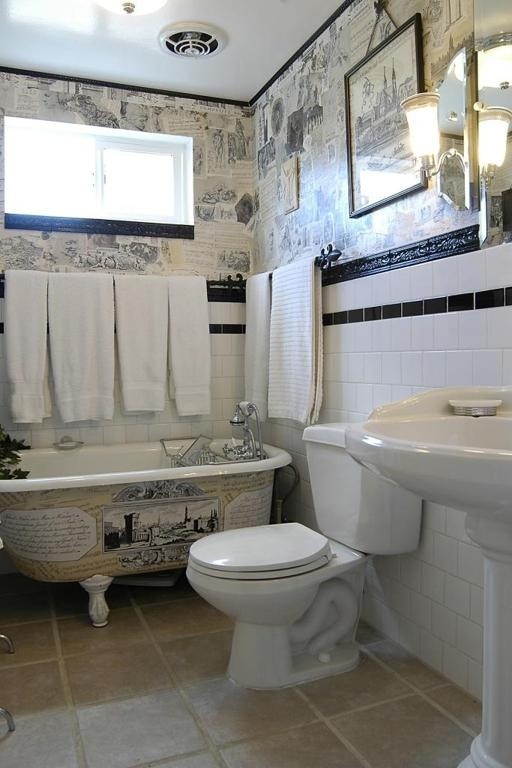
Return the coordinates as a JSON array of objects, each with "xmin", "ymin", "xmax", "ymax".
[{"xmin": 343, "ymin": 386, "xmax": 512, "ymax": 523}]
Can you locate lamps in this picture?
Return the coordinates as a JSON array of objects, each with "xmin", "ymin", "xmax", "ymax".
[
  {"xmin": 473, "ymin": 30, "xmax": 512, "ymax": 191},
  {"xmin": 398, "ymin": 92, "xmax": 466, "ymax": 184}
]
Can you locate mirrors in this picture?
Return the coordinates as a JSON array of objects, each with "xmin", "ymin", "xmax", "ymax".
[{"xmin": 435, "ymin": 31, "xmax": 512, "ymax": 250}]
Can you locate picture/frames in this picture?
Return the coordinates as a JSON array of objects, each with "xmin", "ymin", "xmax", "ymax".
[{"xmin": 343, "ymin": 11, "xmax": 428, "ymax": 220}]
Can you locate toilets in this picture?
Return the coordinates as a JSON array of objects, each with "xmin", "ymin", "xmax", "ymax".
[{"xmin": 184, "ymin": 421, "xmax": 423, "ymax": 688}]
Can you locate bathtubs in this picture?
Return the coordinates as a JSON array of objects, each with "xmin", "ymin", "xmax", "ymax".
[{"xmin": 0, "ymin": 441, "xmax": 294, "ymax": 583}]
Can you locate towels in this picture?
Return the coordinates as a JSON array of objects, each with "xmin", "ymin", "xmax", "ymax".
[
  {"xmin": 243, "ymin": 258, "xmax": 325, "ymax": 427},
  {"xmin": 2, "ymin": 268, "xmax": 212, "ymax": 425}
]
